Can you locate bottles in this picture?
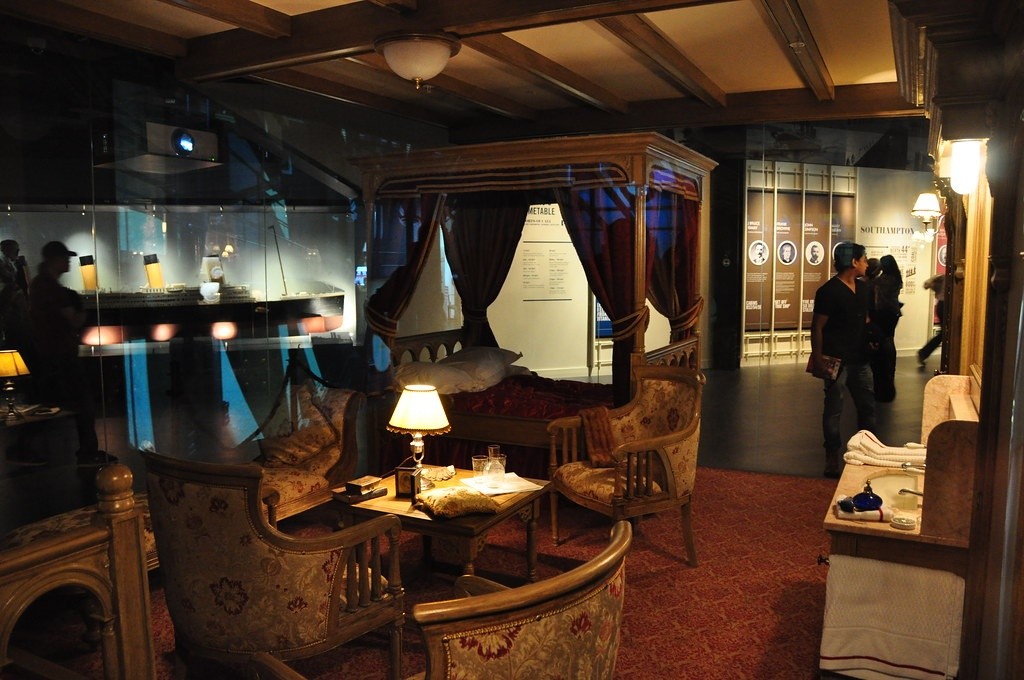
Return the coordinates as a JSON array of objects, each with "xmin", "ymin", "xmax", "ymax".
[{"xmin": 484, "ymin": 445, "xmax": 505, "ymax": 488}]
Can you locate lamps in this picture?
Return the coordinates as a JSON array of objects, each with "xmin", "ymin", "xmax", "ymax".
[
  {"xmin": 373, "ymin": 31, "xmax": 460, "ymax": 90},
  {"xmin": 911, "ymin": 192, "xmax": 947, "ymax": 245},
  {"xmin": 384, "ymin": 384, "xmax": 452, "ymax": 508},
  {"xmin": 1, "ymin": 351, "xmax": 32, "ymax": 421}
]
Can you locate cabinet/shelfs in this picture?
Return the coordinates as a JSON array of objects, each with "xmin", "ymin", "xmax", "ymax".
[{"xmin": 817, "ymin": 374, "xmax": 994, "ymax": 680}]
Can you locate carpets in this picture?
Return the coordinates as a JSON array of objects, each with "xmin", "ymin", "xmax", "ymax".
[{"xmin": 9, "ymin": 464, "xmax": 842, "ymax": 679}]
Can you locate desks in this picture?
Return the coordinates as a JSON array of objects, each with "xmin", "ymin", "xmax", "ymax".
[{"xmin": 1, "ymin": 401, "xmax": 82, "ymax": 528}]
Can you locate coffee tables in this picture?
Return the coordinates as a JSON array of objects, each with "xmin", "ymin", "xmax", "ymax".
[{"xmin": 328, "ymin": 462, "xmax": 555, "ymax": 577}]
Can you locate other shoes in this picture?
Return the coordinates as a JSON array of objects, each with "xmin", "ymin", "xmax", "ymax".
[
  {"xmin": 95, "ymin": 449, "xmax": 122, "ymax": 464},
  {"xmin": 824, "ymin": 450, "xmax": 839, "ymax": 477},
  {"xmin": 917, "ymin": 348, "xmax": 925, "ymax": 366}
]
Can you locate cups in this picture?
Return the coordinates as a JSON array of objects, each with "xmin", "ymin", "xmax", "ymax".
[
  {"xmin": 472, "ymin": 455, "xmax": 488, "ymax": 484},
  {"xmin": 498, "ymin": 454, "xmax": 507, "ymax": 472}
]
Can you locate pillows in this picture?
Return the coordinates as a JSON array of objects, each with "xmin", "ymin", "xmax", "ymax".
[
  {"xmin": 254, "ymin": 382, "xmax": 338, "ymax": 469},
  {"xmin": 385, "ymin": 347, "xmax": 537, "ymax": 395}
]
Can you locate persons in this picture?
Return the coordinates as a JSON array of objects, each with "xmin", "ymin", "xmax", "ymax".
[
  {"xmin": 858, "ymin": 255, "xmax": 903, "ymax": 403},
  {"xmin": 811, "ymin": 242, "xmax": 877, "ymax": 479},
  {"xmin": 752, "ymin": 244, "xmax": 822, "ymax": 264},
  {"xmin": 0, "ymin": 239, "xmax": 34, "ymax": 381},
  {"xmin": 5, "ymin": 241, "xmax": 119, "ymax": 468},
  {"xmin": 917, "ymin": 273, "xmax": 947, "ymax": 365}
]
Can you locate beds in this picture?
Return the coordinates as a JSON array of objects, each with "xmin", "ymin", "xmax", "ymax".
[{"xmin": 343, "ymin": 132, "xmax": 722, "ymax": 455}]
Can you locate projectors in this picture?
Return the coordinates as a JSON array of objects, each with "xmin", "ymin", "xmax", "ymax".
[{"xmin": 92, "ymin": 121, "xmax": 226, "ymax": 176}]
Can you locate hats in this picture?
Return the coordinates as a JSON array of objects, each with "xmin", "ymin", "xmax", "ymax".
[{"xmin": 41, "ymin": 240, "xmax": 78, "ymax": 257}]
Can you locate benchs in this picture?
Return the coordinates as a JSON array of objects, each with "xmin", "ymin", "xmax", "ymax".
[{"xmin": 1, "ymin": 360, "xmax": 367, "ymax": 614}]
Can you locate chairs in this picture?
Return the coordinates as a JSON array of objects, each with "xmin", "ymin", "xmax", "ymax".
[
  {"xmin": 546, "ymin": 366, "xmax": 707, "ymax": 566},
  {"xmin": 122, "ymin": 440, "xmax": 402, "ymax": 680},
  {"xmin": 407, "ymin": 520, "xmax": 635, "ymax": 680}
]
